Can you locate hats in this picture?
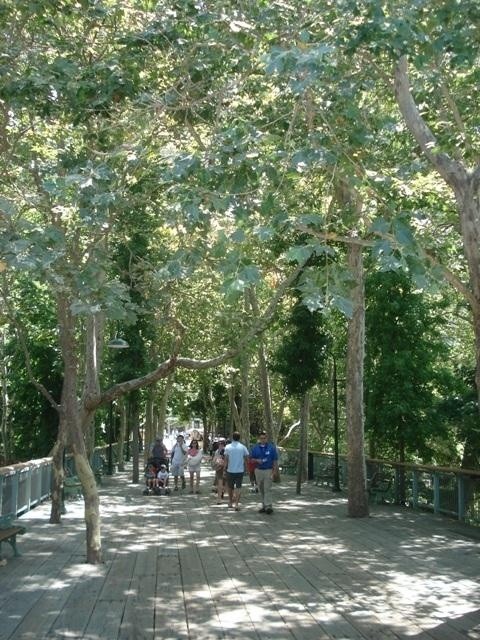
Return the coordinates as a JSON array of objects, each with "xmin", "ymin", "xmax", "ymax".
[
  {"xmin": 160, "ymin": 464, "xmax": 167, "ymax": 469},
  {"xmin": 216, "ymin": 437, "xmax": 226, "ymax": 444}
]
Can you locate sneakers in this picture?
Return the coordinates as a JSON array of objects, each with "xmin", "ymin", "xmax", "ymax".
[
  {"xmin": 250, "ymin": 486, "xmax": 259, "ymax": 493},
  {"xmin": 174, "ymin": 486, "xmax": 202, "ymax": 494},
  {"xmin": 228, "ymin": 503, "xmax": 239, "ymax": 511},
  {"xmin": 258, "ymin": 508, "xmax": 273, "ymax": 514},
  {"xmin": 147, "ymin": 485, "xmax": 165, "ymax": 490}
]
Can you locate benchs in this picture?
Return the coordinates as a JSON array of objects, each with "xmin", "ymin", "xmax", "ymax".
[{"xmin": 63, "ymin": 473, "xmax": 101, "ymax": 502}]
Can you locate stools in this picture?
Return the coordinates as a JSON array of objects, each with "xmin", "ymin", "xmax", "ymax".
[{"xmin": 0, "ymin": 526, "xmax": 27, "ymax": 562}]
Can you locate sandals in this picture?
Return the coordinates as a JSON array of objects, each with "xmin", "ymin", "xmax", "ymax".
[{"xmin": 212, "ymin": 484, "xmax": 224, "ymax": 504}]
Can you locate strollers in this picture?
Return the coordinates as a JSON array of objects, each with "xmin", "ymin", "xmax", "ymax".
[{"xmin": 143, "ymin": 457, "xmax": 170, "ymax": 496}]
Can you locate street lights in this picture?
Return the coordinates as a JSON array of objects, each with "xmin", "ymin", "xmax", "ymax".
[{"xmin": 59, "ymin": 321, "xmax": 131, "ymax": 515}]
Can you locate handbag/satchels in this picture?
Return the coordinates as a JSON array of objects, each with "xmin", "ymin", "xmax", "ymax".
[{"xmin": 213, "ymin": 457, "xmax": 225, "ymax": 472}]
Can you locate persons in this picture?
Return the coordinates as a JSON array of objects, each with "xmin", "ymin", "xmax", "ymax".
[
  {"xmin": 224, "ymin": 431, "xmax": 249, "ymax": 511},
  {"xmin": 157, "ymin": 464, "xmax": 168, "ymax": 488},
  {"xmin": 152, "ymin": 438, "xmax": 167, "ymax": 458},
  {"xmin": 251, "ymin": 432, "xmax": 278, "ymax": 514},
  {"xmin": 208, "ymin": 438, "xmax": 232, "ymax": 505},
  {"xmin": 171, "ymin": 435, "xmax": 188, "ymax": 491},
  {"xmin": 145, "ymin": 464, "xmax": 157, "ymax": 488},
  {"xmin": 246, "ymin": 446, "xmax": 259, "ymax": 493},
  {"xmin": 186, "ymin": 440, "xmax": 204, "ymax": 494}
]
